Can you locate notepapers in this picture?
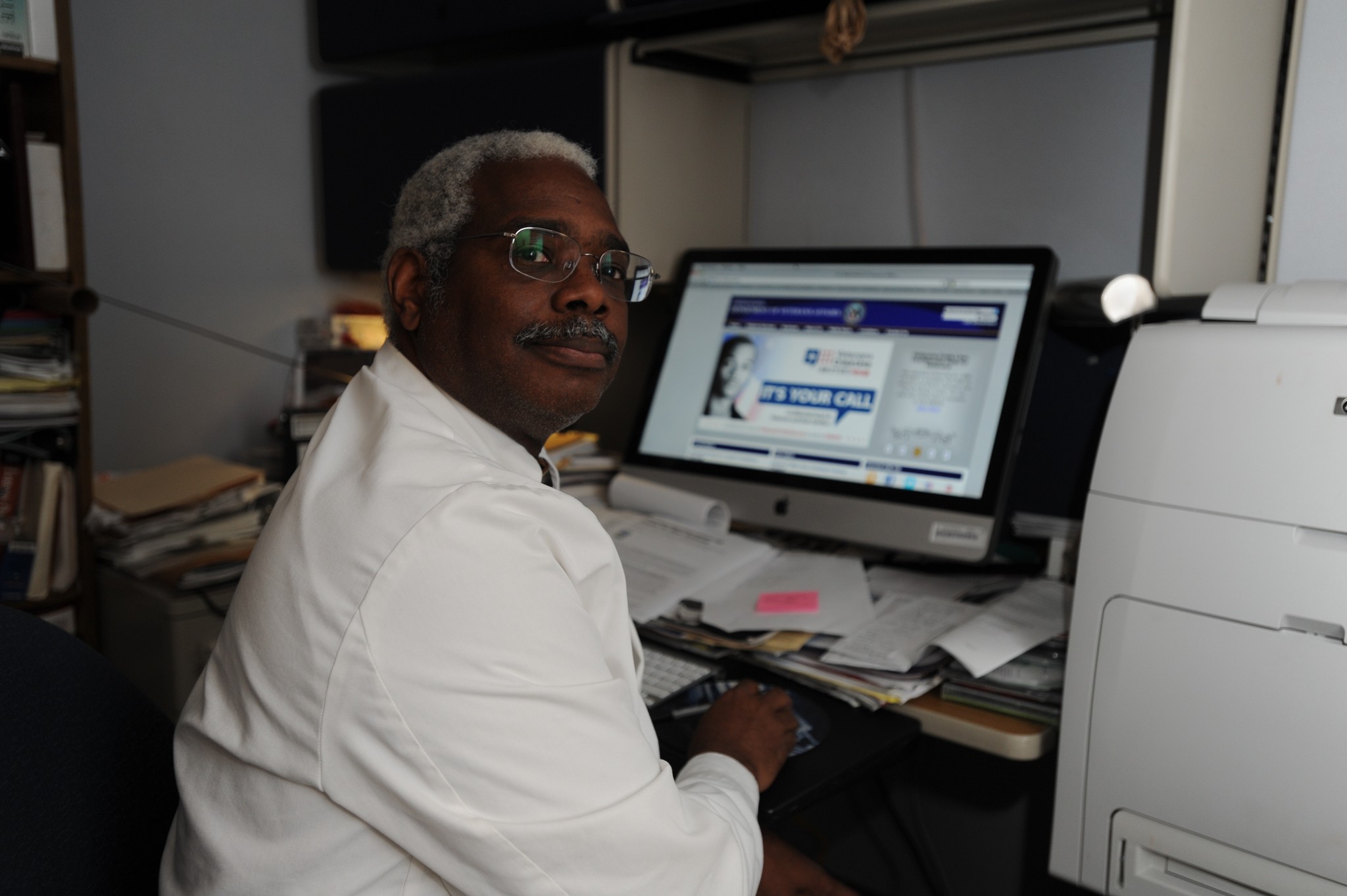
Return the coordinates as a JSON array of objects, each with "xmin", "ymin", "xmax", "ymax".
[{"xmin": 755, "ymin": 589, "xmax": 818, "ymax": 615}]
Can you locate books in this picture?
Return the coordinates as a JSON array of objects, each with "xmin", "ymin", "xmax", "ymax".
[
  {"xmin": 92, "ymin": 443, "xmax": 283, "ymax": 599},
  {"xmin": 0, "ymin": 459, "xmax": 77, "ymax": 600},
  {"xmin": 1, "ymin": 314, "xmax": 82, "ymax": 429},
  {"xmin": 22, "ymin": 136, "xmax": 69, "ymax": 274},
  {"xmin": 0, "ymin": 1, "xmax": 57, "ymax": 60}
]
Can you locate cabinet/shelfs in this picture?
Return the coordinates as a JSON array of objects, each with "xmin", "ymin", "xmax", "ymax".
[{"xmin": 0, "ymin": 0, "xmax": 102, "ymax": 643}]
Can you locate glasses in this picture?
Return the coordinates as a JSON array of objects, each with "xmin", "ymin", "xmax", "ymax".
[{"xmin": 452, "ymin": 226, "xmax": 660, "ymax": 303}]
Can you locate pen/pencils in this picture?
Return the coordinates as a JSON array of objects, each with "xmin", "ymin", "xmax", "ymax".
[{"xmin": 665, "ymin": 699, "xmax": 712, "ymax": 720}]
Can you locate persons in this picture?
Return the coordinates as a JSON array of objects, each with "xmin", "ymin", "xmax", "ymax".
[
  {"xmin": 702, "ymin": 335, "xmax": 759, "ymax": 421},
  {"xmin": 147, "ymin": 127, "xmax": 796, "ymax": 896}
]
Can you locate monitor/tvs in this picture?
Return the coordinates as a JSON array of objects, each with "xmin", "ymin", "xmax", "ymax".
[{"xmin": 622, "ymin": 246, "xmax": 1063, "ymax": 566}]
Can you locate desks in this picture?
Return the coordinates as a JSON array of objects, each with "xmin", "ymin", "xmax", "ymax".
[{"xmin": 565, "ymin": 486, "xmax": 1061, "ymax": 766}]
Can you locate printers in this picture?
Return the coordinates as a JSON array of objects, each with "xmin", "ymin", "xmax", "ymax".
[{"xmin": 1046, "ymin": 288, "xmax": 1347, "ymax": 896}]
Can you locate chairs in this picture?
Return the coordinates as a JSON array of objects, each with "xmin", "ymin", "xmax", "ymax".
[{"xmin": 0, "ymin": 606, "xmax": 177, "ymax": 896}]
